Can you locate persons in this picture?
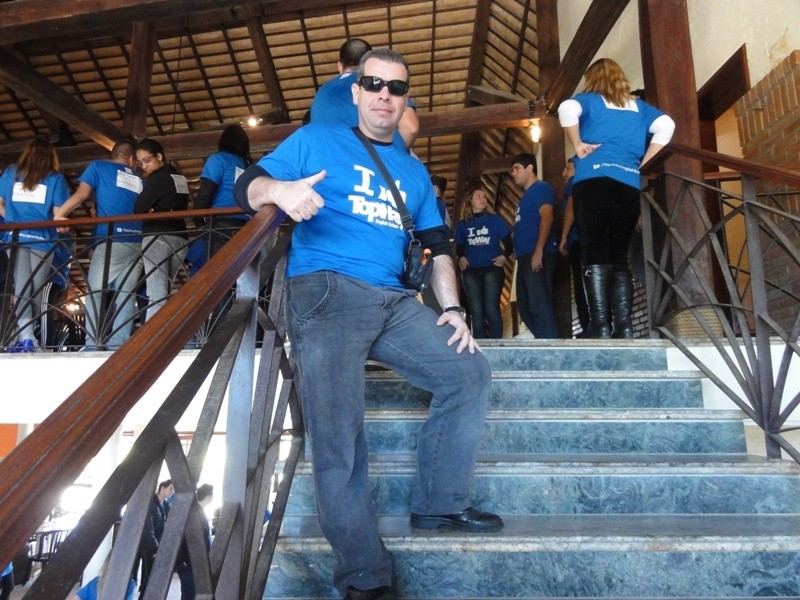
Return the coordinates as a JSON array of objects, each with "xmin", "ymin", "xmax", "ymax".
[
  {"xmin": 556, "ymin": 58, "xmax": 676, "ymax": 340},
  {"xmin": 508, "ymin": 153, "xmax": 613, "ymax": 338},
  {"xmin": 309, "ymin": 38, "xmax": 420, "ymax": 370},
  {"xmin": 453, "ymin": 187, "xmax": 513, "ymax": 339},
  {"xmin": 0, "ymin": 125, "xmax": 265, "ymax": 352},
  {"xmin": 0, "ymin": 479, "xmax": 215, "ymax": 600},
  {"xmin": 417, "ymin": 174, "xmax": 452, "ymax": 315},
  {"xmin": 233, "ymin": 47, "xmax": 504, "ymax": 600}
]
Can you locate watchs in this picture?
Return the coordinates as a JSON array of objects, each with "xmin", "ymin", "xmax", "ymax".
[{"xmin": 445, "ymin": 306, "xmax": 466, "ymax": 322}]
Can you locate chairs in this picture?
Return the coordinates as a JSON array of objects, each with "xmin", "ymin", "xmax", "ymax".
[{"xmin": 22, "ymin": 530, "xmax": 67, "ymax": 585}]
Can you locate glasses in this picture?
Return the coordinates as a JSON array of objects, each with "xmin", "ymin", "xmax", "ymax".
[
  {"xmin": 357, "ymin": 75, "xmax": 407, "ymax": 96},
  {"xmin": 136, "ymin": 155, "xmax": 154, "ymax": 167}
]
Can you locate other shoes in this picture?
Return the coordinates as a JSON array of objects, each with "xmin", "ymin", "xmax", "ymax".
[
  {"xmin": 78, "ymin": 346, "xmax": 97, "ymax": 351},
  {"xmin": 18, "ymin": 336, "xmax": 43, "ymax": 352}
]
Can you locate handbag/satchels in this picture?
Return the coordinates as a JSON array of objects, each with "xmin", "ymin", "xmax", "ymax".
[{"xmin": 402, "ymin": 239, "xmax": 433, "ymax": 289}]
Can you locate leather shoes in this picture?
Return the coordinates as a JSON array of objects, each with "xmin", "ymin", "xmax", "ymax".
[
  {"xmin": 411, "ymin": 507, "xmax": 504, "ymax": 532},
  {"xmin": 344, "ymin": 587, "xmax": 396, "ymax": 600}
]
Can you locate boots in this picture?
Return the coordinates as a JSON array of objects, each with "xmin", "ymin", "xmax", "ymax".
[
  {"xmin": 610, "ymin": 271, "xmax": 634, "ymax": 339},
  {"xmin": 574, "ymin": 263, "xmax": 611, "ymax": 338}
]
